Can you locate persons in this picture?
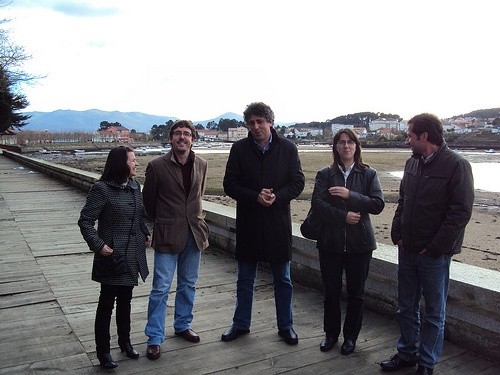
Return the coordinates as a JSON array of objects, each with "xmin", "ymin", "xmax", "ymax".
[
  {"xmin": 222, "ymin": 102, "xmax": 305, "ymax": 345},
  {"xmin": 78, "ymin": 147, "xmax": 152, "ymax": 369},
  {"xmin": 380, "ymin": 113, "xmax": 474, "ymax": 375},
  {"xmin": 141, "ymin": 121, "xmax": 210, "ymax": 359},
  {"xmin": 311, "ymin": 128, "xmax": 385, "ymax": 355}
]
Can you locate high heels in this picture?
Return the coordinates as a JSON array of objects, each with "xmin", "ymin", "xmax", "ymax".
[
  {"xmin": 97, "ymin": 354, "xmax": 118, "ymax": 369},
  {"xmin": 119, "ymin": 342, "xmax": 139, "ymax": 358}
]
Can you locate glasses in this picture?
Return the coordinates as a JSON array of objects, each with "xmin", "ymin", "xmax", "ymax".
[{"xmin": 173, "ymin": 132, "xmax": 192, "ymax": 137}]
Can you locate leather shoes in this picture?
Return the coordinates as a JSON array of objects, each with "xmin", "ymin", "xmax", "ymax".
[
  {"xmin": 341, "ymin": 338, "xmax": 356, "ymax": 355},
  {"xmin": 380, "ymin": 353, "xmax": 417, "ymax": 371},
  {"xmin": 416, "ymin": 364, "xmax": 433, "ymax": 375},
  {"xmin": 176, "ymin": 328, "xmax": 200, "ymax": 343},
  {"xmin": 320, "ymin": 335, "xmax": 338, "ymax": 352},
  {"xmin": 221, "ymin": 325, "xmax": 250, "ymax": 341},
  {"xmin": 146, "ymin": 345, "xmax": 161, "ymax": 360},
  {"xmin": 278, "ymin": 328, "xmax": 298, "ymax": 345}
]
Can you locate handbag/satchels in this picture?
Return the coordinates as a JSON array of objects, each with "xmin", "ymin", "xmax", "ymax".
[
  {"xmin": 300, "ymin": 166, "xmax": 332, "ymax": 240},
  {"xmin": 93, "ymin": 255, "xmax": 129, "ymax": 279}
]
